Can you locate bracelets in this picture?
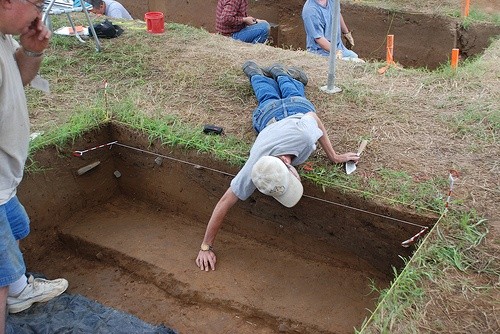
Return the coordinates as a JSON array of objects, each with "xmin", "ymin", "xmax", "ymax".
[
  {"xmin": 201, "ymin": 245, "xmax": 212, "ymax": 251},
  {"xmin": 24, "ymin": 50, "xmax": 43, "ymax": 57}
]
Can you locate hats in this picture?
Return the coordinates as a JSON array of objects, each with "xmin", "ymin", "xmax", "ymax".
[{"xmin": 253, "ymin": 155, "xmax": 304, "ymax": 209}]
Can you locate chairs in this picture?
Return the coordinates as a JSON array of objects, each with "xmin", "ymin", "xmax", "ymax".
[{"xmin": 41, "ymin": 0, "xmax": 104, "ymax": 51}]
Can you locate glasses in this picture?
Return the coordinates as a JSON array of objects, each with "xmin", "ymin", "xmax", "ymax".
[{"xmin": 21, "ymin": 0, "xmax": 45, "ymax": 12}]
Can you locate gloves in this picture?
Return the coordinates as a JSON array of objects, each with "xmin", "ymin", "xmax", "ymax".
[{"xmin": 343, "ymin": 33, "xmax": 355, "ymax": 50}]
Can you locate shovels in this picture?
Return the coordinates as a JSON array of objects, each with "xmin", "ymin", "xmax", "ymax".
[{"xmin": 346, "ymin": 140, "xmax": 367, "ymax": 174}]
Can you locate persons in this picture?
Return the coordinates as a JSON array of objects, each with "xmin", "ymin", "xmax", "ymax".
[
  {"xmin": 87, "ymin": 0, "xmax": 133, "ymax": 20},
  {"xmin": 216, "ymin": 0, "xmax": 271, "ymax": 44},
  {"xmin": 195, "ymin": 61, "xmax": 360, "ymax": 272},
  {"xmin": 302, "ymin": 0, "xmax": 358, "ymax": 59},
  {"xmin": 0, "ymin": 0, "xmax": 69, "ymax": 334}
]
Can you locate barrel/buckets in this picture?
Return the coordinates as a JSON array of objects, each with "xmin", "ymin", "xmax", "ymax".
[{"xmin": 145, "ymin": 11, "xmax": 164, "ymax": 33}]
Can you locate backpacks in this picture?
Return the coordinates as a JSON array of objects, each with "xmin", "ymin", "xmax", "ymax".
[{"xmin": 88, "ymin": 20, "xmax": 123, "ymax": 39}]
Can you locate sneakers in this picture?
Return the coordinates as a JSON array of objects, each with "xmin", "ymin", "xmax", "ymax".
[
  {"xmin": 269, "ymin": 64, "xmax": 308, "ymax": 86},
  {"xmin": 6, "ymin": 273, "xmax": 69, "ymax": 314},
  {"xmin": 242, "ymin": 61, "xmax": 267, "ymax": 75}
]
